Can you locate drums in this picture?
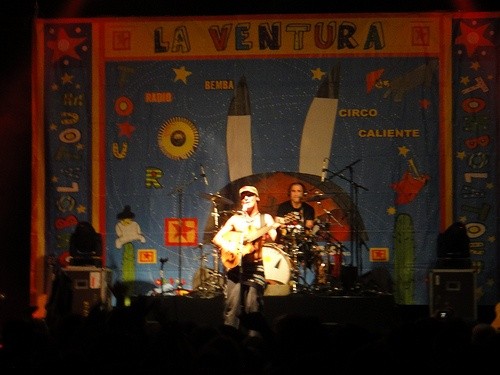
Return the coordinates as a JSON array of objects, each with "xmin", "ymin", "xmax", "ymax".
[{"xmin": 261, "ymin": 243, "xmax": 300, "ymax": 295}]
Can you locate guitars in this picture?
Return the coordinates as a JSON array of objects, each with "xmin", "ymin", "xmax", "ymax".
[{"xmin": 220, "ymin": 212, "xmax": 301, "ymax": 269}]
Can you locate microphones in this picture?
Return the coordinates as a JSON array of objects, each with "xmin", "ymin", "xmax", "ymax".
[
  {"xmin": 200, "ymin": 164, "xmax": 208, "ymax": 186},
  {"xmin": 321, "ymin": 159, "xmax": 326, "ymax": 182}
]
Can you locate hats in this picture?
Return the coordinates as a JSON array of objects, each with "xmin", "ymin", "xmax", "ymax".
[{"xmin": 239, "ymin": 186, "xmax": 259, "ymax": 198}]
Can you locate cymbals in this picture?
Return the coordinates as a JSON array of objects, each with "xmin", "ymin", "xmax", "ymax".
[{"xmin": 300, "ymin": 193, "xmax": 328, "ymax": 203}]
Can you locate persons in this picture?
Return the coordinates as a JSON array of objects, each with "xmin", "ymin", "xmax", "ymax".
[
  {"xmin": 0, "ymin": 277, "xmax": 500, "ymax": 375},
  {"xmin": 212, "ymin": 186, "xmax": 276, "ymax": 337},
  {"xmin": 274, "ymin": 183, "xmax": 316, "ymax": 286}
]
